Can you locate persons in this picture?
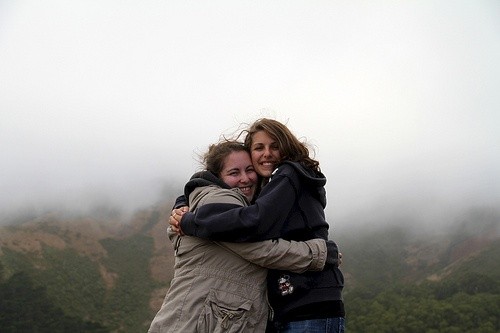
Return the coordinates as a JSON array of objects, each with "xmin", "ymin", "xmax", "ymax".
[
  {"xmin": 169, "ymin": 118, "xmax": 345, "ymax": 333},
  {"xmin": 147, "ymin": 140, "xmax": 342, "ymax": 333}
]
